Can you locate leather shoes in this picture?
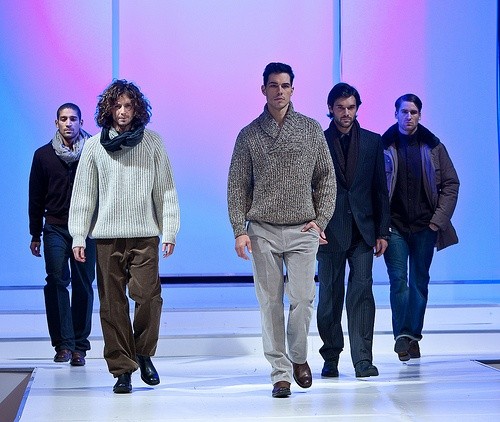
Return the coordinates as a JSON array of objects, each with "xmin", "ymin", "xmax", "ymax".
[
  {"xmin": 138, "ymin": 355, "xmax": 160, "ymax": 385},
  {"xmin": 291, "ymin": 361, "xmax": 312, "ymax": 388},
  {"xmin": 113, "ymin": 373, "xmax": 132, "ymax": 394},
  {"xmin": 355, "ymin": 360, "xmax": 378, "ymax": 377},
  {"xmin": 409, "ymin": 339, "xmax": 420, "ymax": 358},
  {"xmin": 321, "ymin": 360, "xmax": 340, "ymax": 377},
  {"xmin": 70, "ymin": 352, "xmax": 86, "ymax": 366},
  {"xmin": 271, "ymin": 380, "xmax": 292, "ymax": 397},
  {"xmin": 395, "ymin": 338, "xmax": 411, "ymax": 362},
  {"xmin": 54, "ymin": 349, "xmax": 72, "ymax": 362}
]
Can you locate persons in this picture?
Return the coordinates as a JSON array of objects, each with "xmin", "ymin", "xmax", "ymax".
[
  {"xmin": 68, "ymin": 78, "xmax": 179, "ymax": 393},
  {"xmin": 316, "ymin": 82, "xmax": 391, "ymax": 377},
  {"xmin": 381, "ymin": 94, "xmax": 459, "ymax": 361},
  {"xmin": 28, "ymin": 103, "xmax": 95, "ymax": 366},
  {"xmin": 227, "ymin": 63, "xmax": 337, "ymax": 397}
]
